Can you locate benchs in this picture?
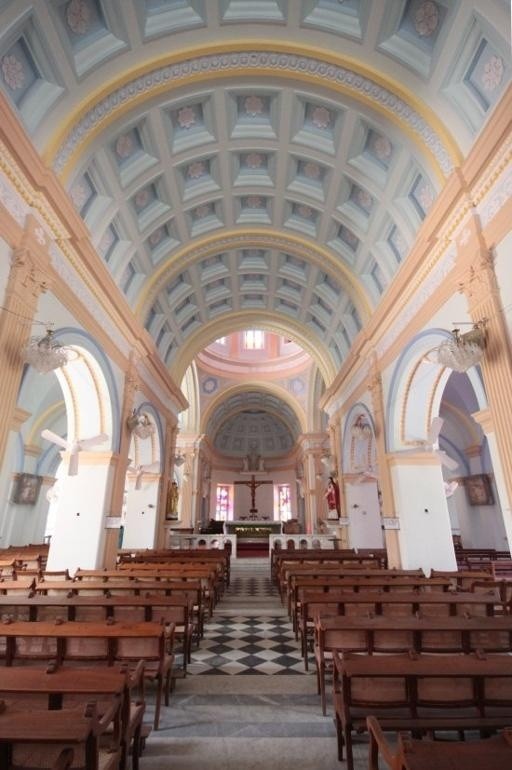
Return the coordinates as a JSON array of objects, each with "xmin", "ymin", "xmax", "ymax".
[
  {"xmin": 271, "ymin": 533, "xmax": 510, "ymax": 766},
  {"xmin": 2, "ymin": 547, "xmax": 237, "ymax": 768}
]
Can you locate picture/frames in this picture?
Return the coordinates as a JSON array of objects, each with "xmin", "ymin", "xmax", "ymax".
[
  {"xmin": 461, "ymin": 472, "xmax": 494, "ymax": 507},
  {"xmin": 13, "ymin": 471, "xmax": 41, "ymax": 505}
]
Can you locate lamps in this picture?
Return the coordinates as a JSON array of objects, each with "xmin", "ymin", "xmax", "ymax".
[
  {"xmin": 21, "ymin": 313, "xmax": 67, "ymax": 375},
  {"xmin": 128, "ymin": 408, "xmax": 157, "ymax": 439},
  {"xmin": 435, "ymin": 314, "xmax": 491, "ymax": 372},
  {"xmin": 350, "ymin": 414, "xmax": 373, "ymax": 443}
]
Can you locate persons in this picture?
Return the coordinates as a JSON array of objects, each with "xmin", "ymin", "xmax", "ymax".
[
  {"xmin": 169, "ymin": 482, "xmax": 179, "ymax": 514},
  {"xmin": 246, "ymin": 481, "xmax": 260, "ymax": 507},
  {"xmin": 322, "ymin": 476, "xmax": 338, "ymax": 520}
]
